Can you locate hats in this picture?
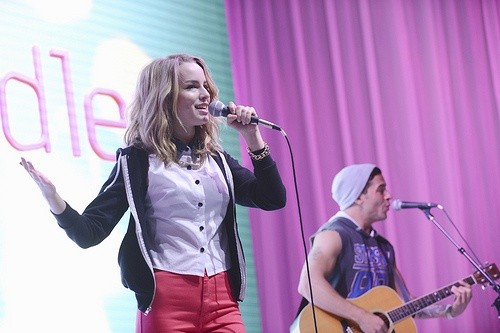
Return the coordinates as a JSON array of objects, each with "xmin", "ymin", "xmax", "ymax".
[{"xmin": 330, "ymin": 163, "xmax": 377, "ymax": 212}]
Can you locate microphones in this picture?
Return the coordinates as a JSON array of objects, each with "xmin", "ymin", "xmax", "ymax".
[
  {"xmin": 391, "ymin": 199, "xmax": 437, "ymax": 210},
  {"xmin": 208, "ymin": 101, "xmax": 281, "ymax": 131}
]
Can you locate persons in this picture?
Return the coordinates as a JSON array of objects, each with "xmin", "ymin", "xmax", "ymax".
[
  {"xmin": 294, "ymin": 164, "xmax": 473, "ymax": 333},
  {"xmin": 20, "ymin": 55, "xmax": 288, "ymax": 333}
]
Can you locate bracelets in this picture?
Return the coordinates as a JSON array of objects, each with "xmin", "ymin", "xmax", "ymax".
[
  {"xmin": 248, "ymin": 144, "xmax": 270, "ymax": 160},
  {"xmin": 447, "ymin": 305, "xmax": 454, "ymax": 319}
]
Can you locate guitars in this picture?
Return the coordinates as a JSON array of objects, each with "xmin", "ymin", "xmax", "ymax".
[{"xmin": 289, "ymin": 261, "xmax": 500, "ymax": 333}]
